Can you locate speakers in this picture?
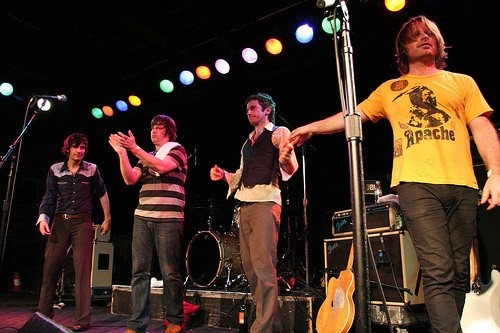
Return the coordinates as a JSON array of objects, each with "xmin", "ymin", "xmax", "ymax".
[
  {"xmin": 324, "ymin": 230, "xmax": 425, "ymax": 306},
  {"xmin": 18, "ymin": 312, "xmax": 72, "ymax": 333}
]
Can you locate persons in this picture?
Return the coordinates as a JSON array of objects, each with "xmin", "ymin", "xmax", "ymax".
[
  {"xmin": 289, "ymin": 15, "xmax": 500, "ymax": 333},
  {"xmin": 210, "ymin": 93, "xmax": 299, "ymax": 333},
  {"xmin": 108, "ymin": 115, "xmax": 188, "ymax": 333},
  {"xmin": 35, "ymin": 133, "xmax": 112, "ymax": 330}
]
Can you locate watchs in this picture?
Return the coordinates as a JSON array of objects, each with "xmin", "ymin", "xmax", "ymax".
[{"xmin": 486, "ymin": 167, "xmax": 500, "ymax": 177}]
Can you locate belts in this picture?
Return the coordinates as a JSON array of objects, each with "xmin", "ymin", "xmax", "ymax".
[{"xmin": 57, "ymin": 212, "xmax": 79, "ymax": 219}]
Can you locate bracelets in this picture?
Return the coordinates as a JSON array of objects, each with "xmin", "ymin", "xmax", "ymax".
[{"xmin": 131, "ymin": 145, "xmax": 142, "ymax": 155}]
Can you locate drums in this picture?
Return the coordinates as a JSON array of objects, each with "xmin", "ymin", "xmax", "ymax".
[{"xmin": 185, "ymin": 231, "xmax": 243, "ymax": 287}]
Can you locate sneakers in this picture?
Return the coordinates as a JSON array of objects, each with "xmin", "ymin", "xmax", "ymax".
[
  {"xmin": 165, "ymin": 324, "xmax": 181, "ymax": 333},
  {"xmin": 124, "ymin": 329, "xmax": 136, "ymax": 333}
]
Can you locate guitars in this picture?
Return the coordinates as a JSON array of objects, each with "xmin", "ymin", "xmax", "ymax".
[{"xmin": 316, "ymin": 241, "xmax": 355, "ymax": 333}]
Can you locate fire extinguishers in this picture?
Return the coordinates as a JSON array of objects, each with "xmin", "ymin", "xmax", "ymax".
[{"xmin": 13, "ymin": 272, "xmax": 21, "ymax": 291}]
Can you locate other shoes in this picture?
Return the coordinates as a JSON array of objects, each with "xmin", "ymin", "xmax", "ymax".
[{"xmin": 72, "ymin": 324, "xmax": 86, "ymax": 332}]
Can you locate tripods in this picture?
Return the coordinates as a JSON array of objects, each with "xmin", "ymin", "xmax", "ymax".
[{"xmin": 275, "ymin": 146, "xmax": 323, "ymax": 297}]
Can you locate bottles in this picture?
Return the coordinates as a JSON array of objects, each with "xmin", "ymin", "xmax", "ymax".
[
  {"xmin": 394, "ymin": 204, "xmax": 407, "ymax": 231},
  {"xmin": 374, "ymin": 181, "xmax": 382, "ymax": 205},
  {"xmin": 238, "ymin": 297, "xmax": 249, "ymax": 333}
]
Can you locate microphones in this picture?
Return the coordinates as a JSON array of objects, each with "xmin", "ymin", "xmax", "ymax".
[
  {"xmin": 34, "ymin": 95, "xmax": 67, "ymax": 103},
  {"xmin": 316, "ymin": 0, "xmax": 336, "ymax": 9}
]
